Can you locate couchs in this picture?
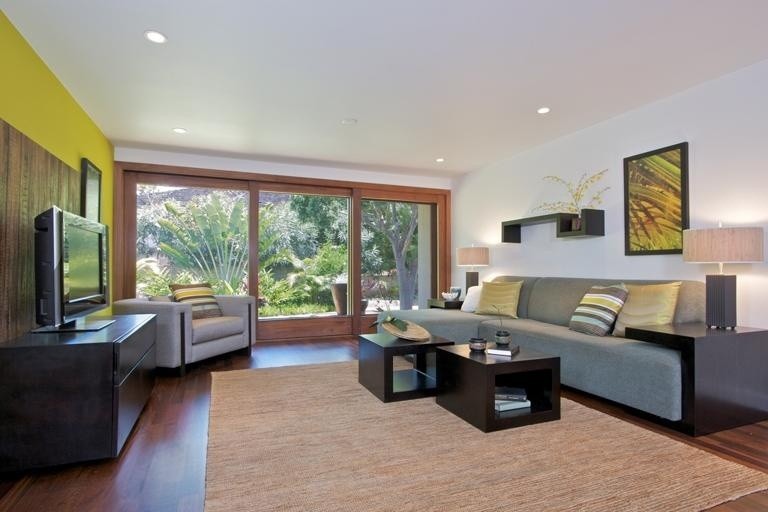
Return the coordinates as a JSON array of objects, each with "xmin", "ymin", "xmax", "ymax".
[
  {"xmin": 111, "ymin": 295, "xmax": 255, "ymax": 378},
  {"xmin": 377, "ymin": 276, "xmax": 539, "ymax": 364},
  {"xmin": 479, "ymin": 277, "xmax": 706, "ymax": 422}
]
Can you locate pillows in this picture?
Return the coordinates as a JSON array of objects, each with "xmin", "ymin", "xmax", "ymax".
[
  {"xmin": 169, "ymin": 283, "xmax": 222, "ymax": 319},
  {"xmin": 461, "ymin": 286, "xmax": 483, "ymax": 312},
  {"xmin": 569, "ymin": 285, "xmax": 628, "ymax": 337},
  {"xmin": 612, "ymin": 282, "xmax": 681, "ymax": 338},
  {"xmin": 475, "ymin": 281, "xmax": 524, "ymax": 319}
]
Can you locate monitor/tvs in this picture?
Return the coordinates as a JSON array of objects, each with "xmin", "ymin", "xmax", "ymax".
[{"xmin": 29, "ymin": 206, "xmax": 116, "ymax": 333}]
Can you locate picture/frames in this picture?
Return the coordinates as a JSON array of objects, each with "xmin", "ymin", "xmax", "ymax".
[
  {"xmin": 80, "ymin": 158, "xmax": 102, "ymax": 223},
  {"xmin": 623, "ymin": 142, "xmax": 690, "ymax": 256}
]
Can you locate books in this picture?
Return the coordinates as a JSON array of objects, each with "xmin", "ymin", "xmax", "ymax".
[
  {"xmin": 495, "ymin": 386, "xmax": 527, "ymax": 402},
  {"xmin": 495, "ymin": 399, "xmax": 531, "ymax": 411},
  {"xmin": 487, "ymin": 344, "xmax": 520, "ymax": 356}
]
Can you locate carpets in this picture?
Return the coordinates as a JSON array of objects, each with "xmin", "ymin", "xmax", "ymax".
[{"xmin": 205, "ymin": 355, "xmax": 768, "ymax": 512}]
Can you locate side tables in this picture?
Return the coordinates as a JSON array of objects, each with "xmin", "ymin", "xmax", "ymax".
[
  {"xmin": 427, "ymin": 299, "xmax": 463, "ymax": 309},
  {"xmin": 625, "ymin": 323, "xmax": 767, "ymax": 437}
]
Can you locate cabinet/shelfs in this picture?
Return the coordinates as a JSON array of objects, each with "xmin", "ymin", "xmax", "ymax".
[{"xmin": 0, "ymin": 314, "xmax": 157, "ymax": 483}]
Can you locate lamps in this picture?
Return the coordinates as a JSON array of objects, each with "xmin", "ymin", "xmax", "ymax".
[
  {"xmin": 683, "ymin": 223, "xmax": 764, "ymax": 330},
  {"xmin": 456, "ymin": 247, "xmax": 489, "ymax": 296}
]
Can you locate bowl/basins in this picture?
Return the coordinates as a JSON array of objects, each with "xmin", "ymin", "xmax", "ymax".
[{"xmin": 442, "ymin": 292, "xmax": 459, "ymax": 300}]
